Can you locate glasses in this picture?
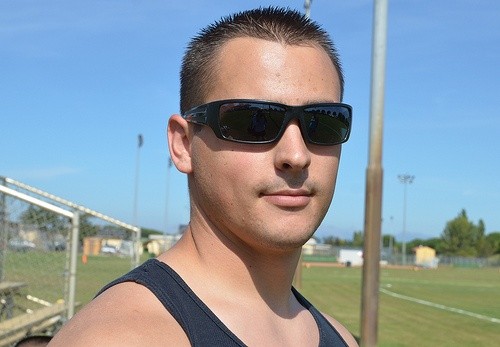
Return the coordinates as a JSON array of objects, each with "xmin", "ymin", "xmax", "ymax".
[{"xmin": 183, "ymin": 98, "xmax": 354, "ymax": 144}]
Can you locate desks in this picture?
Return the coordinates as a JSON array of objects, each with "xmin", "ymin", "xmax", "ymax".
[{"xmin": 0, "ymin": 282, "xmax": 28, "ymax": 319}]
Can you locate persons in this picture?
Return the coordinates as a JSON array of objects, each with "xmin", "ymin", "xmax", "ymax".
[
  {"xmin": 46, "ymin": 4, "xmax": 362, "ymax": 347},
  {"xmin": 14, "ymin": 335, "xmax": 55, "ymax": 347}
]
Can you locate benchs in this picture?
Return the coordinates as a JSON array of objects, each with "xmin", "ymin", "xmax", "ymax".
[{"xmin": 0, "ymin": 299, "xmax": 82, "ymax": 347}]
